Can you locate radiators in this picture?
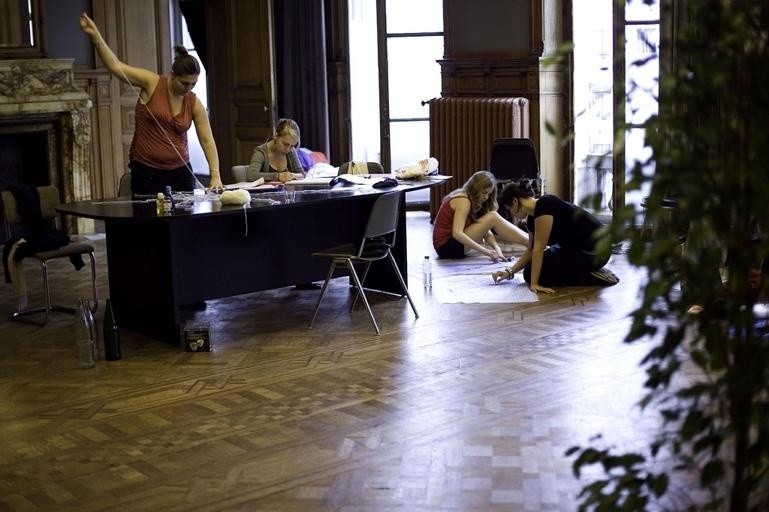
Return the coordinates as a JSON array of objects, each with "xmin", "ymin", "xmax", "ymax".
[{"xmin": 428, "ymin": 97, "xmax": 529, "ymax": 224}]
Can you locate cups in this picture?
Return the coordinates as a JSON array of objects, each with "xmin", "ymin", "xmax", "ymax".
[{"xmin": 283, "ymin": 184, "xmax": 296, "ymax": 202}]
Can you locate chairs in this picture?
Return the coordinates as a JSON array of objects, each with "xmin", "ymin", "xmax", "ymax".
[
  {"xmin": 304, "ymin": 190, "xmax": 419, "ymax": 335},
  {"xmin": 0, "ymin": 185, "xmax": 105, "ymax": 326},
  {"xmin": 115, "ymin": 161, "xmax": 386, "ymax": 196},
  {"xmin": 490, "ymin": 137, "xmax": 541, "ymax": 197},
  {"xmin": 636, "ymin": 195, "xmax": 688, "ymax": 260}
]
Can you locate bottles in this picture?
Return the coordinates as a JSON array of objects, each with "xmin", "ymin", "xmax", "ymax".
[
  {"xmin": 73, "ymin": 296, "xmax": 122, "ymax": 368},
  {"xmin": 155, "ymin": 185, "xmax": 174, "ymax": 215},
  {"xmin": 422, "ymin": 256, "xmax": 433, "ymax": 289}
]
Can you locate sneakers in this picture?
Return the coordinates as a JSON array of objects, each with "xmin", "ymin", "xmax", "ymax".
[{"xmin": 588, "ymin": 267, "xmax": 619, "ymax": 285}]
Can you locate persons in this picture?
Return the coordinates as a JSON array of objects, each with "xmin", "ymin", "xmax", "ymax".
[
  {"xmin": 80, "ymin": 10, "xmax": 224, "ymax": 197},
  {"xmin": 247, "ymin": 118, "xmax": 306, "ymax": 182},
  {"xmin": 433, "ymin": 170, "xmax": 549, "ymax": 264},
  {"xmin": 492, "ymin": 177, "xmax": 619, "ymax": 295}
]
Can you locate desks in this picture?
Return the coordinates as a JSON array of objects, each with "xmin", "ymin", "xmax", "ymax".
[{"xmin": 54, "ymin": 173, "xmax": 453, "ymax": 346}]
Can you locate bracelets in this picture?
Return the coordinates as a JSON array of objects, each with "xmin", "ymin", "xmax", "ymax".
[
  {"xmin": 210, "ymin": 169, "xmax": 219, "ymax": 171},
  {"xmin": 505, "ymin": 268, "xmax": 514, "ymax": 280}
]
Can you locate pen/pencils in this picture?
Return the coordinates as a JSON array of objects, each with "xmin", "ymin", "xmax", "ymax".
[{"xmin": 284, "ymin": 166, "xmax": 288, "ymax": 172}]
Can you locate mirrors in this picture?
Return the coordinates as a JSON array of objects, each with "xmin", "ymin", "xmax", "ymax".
[{"xmin": 0, "ymin": 0, "xmax": 49, "ymax": 59}]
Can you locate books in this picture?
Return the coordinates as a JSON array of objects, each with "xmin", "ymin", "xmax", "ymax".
[{"xmin": 219, "ymin": 162, "xmax": 373, "ymax": 191}]
